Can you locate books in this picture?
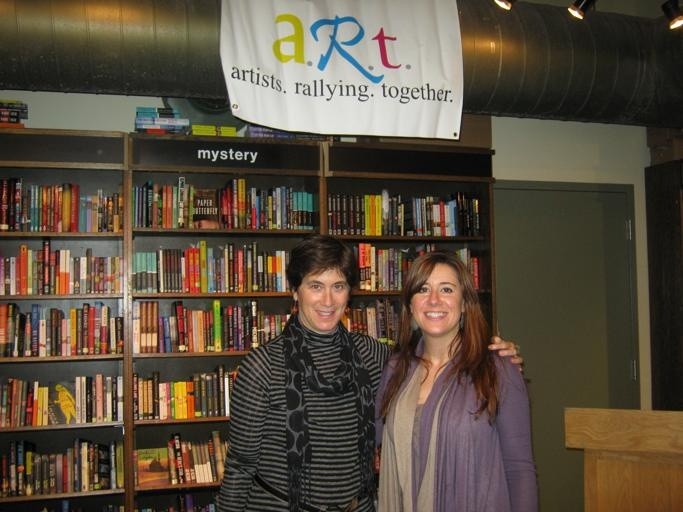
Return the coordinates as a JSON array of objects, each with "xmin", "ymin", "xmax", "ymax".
[
  {"xmin": 0, "ymin": 240, "xmax": 123, "ymax": 296},
  {"xmin": 0, "ymin": 374, "xmax": 123, "ymax": 428},
  {"xmin": 0, "ymin": 437, "xmax": 123, "ymax": 498},
  {"xmin": 0, "ymin": 96, "xmax": 32, "ymax": 132},
  {"xmin": 1, "ymin": 176, "xmax": 124, "ymax": 232},
  {"xmin": 133, "ymin": 431, "xmax": 230, "ymax": 487},
  {"xmin": 129, "ymin": 104, "xmax": 370, "ymax": 142},
  {"xmin": 290, "ymin": 183, "xmax": 483, "ymax": 354},
  {"xmin": 132, "ymin": 240, "xmax": 290, "ymax": 295},
  {"xmin": 134, "ymin": 177, "xmax": 291, "ymax": 231},
  {"xmin": 0, "ymin": 301, "xmax": 124, "ymax": 357},
  {"xmin": 44, "ymin": 500, "xmax": 124, "ymax": 511},
  {"xmin": 133, "ymin": 299, "xmax": 290, "ymax": 354},
  {"xmin": 138, "ymin": 494, "xmax": 215, "ymax": 511},
  {"xmin": 133, "ymin": 365, "xmax": 239, "ymax": 421}
]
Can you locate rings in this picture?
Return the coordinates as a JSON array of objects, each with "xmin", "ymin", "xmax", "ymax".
[{"xmin": 513, "ymin": 344, "xmax": 520, "ymax": 355}]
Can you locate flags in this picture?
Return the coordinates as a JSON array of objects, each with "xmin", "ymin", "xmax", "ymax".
[{"xmin": 217, "ymin": 0, "xmax": 463, "ymax": 142}]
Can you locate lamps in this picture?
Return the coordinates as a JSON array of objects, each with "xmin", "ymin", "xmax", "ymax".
[
  {"xmin": 493, "ymin": 0, "xmax": 516, "ymax": 11},
  {"xmin": 568, "ymin": 0, "xmax": 597, "ymax": 20},
  {"xmin": 661, "ymin": 0, "xmax": 683, "ymax": 30}
]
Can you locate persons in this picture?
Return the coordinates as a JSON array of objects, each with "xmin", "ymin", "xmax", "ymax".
[
  {"xmin": 371, "ymin": 249, "xmax": 542, "ymax": 512},
  {"xmin": 212, "ymin": 234, "xmax": 525, "ymax": 512}
]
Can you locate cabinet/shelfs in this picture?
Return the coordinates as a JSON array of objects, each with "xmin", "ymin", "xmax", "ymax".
[
  {"xmin": 328, "ymin": 129, "xmax": 497, "ymax": 468},
  {"xmin": 1, "ymin": 125, "xmax": 127, "ymax": 510},
  {"xmin": 133, "ymin": 128, "xmax": 328, "ymax": 510}
]
